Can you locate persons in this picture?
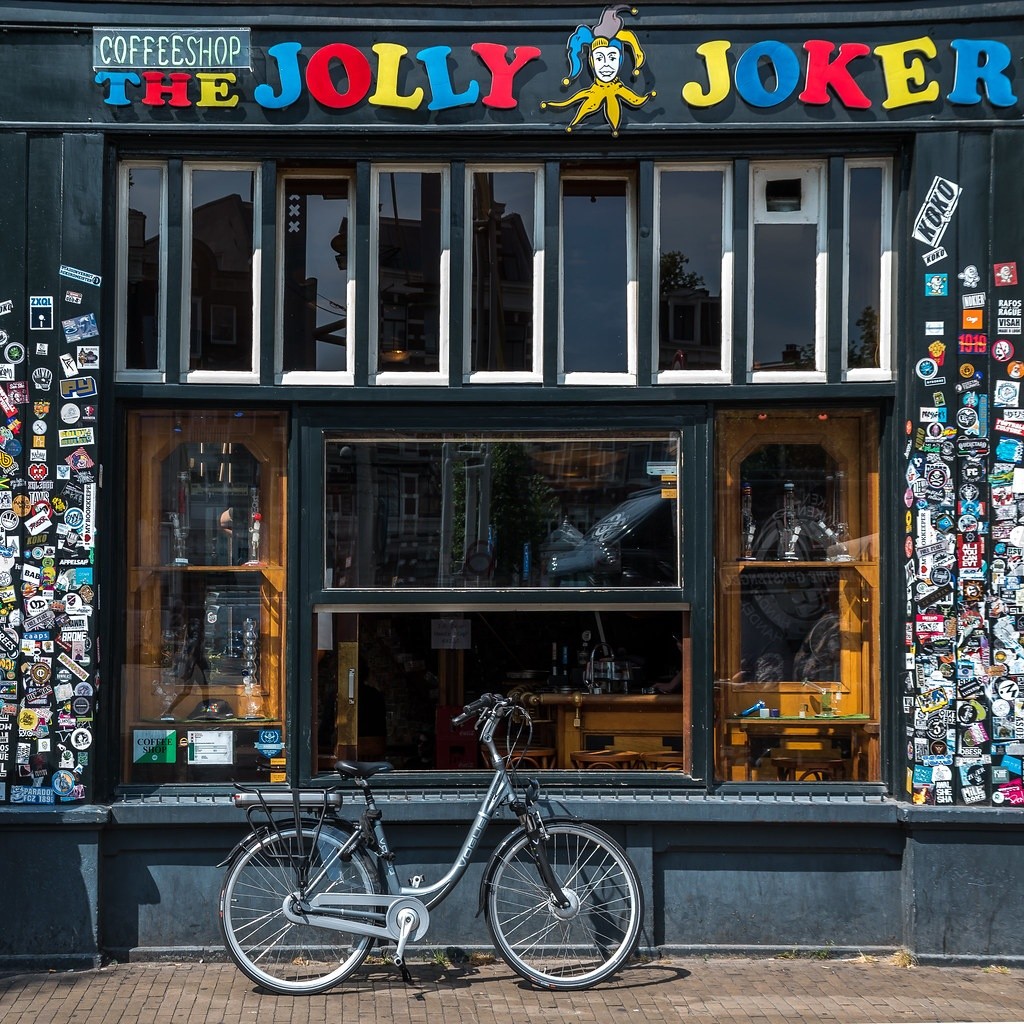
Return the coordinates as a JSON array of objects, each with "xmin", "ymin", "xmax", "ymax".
[{"xmin": 162, "ymin": 607, "xmax": 210, "ymax": 728}]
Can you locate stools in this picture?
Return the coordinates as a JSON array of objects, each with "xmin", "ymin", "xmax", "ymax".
[{"xmin": 481, "ymin": 743, "xmax": 844, "ymax": 782}]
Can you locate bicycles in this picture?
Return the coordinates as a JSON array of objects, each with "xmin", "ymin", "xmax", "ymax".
[{"xmin": 212, "ymin": 690, "xmax": 647, "ymax": 998}]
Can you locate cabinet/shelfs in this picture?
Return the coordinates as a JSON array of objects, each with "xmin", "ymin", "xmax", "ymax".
[
  {"xmin": 713, "ymin": 406, "xmax": 881, "ymax": 781},
  {"xmin": 124, "ymin": 409, "xmax": 287, "ymax": 782}
]
